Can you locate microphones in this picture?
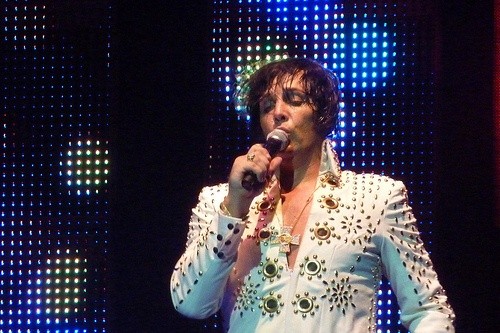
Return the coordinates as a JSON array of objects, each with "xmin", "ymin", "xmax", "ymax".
[{"xmin": 241, "ymin": 128, "xmax": 289, "ymax": 190}]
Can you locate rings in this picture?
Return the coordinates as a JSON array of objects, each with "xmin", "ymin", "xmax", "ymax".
[{"xmin": 247, "ymin": 152, "xmax": 256, "ymax": 161}]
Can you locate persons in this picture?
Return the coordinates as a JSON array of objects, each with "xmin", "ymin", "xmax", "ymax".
[{"xmin": 169, "ymin": 57, "xmax": 456, "ymax": 333}]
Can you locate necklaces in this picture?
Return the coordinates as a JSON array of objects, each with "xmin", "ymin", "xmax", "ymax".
[{"xmin": 266, "ymin": 177, "xmax": 324, "ymax": 253}]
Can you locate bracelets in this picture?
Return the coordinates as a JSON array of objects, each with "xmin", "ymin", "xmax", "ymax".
[{"xmin": 219, "ymin": 201, "xmax": 251, "ymax": 221}]
